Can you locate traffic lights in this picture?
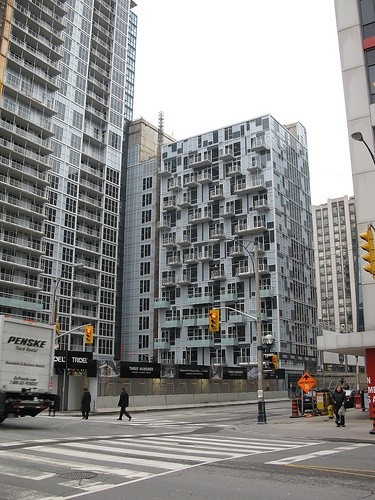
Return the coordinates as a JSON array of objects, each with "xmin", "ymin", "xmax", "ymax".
[
  {"xmin": 85, "ymin": 325, "xmax": 94, "ymax": 344},
  {"xmin": 267, "ymin": 355, "xmax": 279, "ymax": 370},
  {"xmin": 209, "ymin": 309, "xmax": 219, "ymax": 332},
  {"xmin": 358, "ymin": 228, "xmax": 375, "ymax": 276}
]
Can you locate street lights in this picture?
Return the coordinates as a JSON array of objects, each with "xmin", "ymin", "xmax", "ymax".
[
  {"xmin": 52, "ymin": 263, "xmax": 84, "ymax": 326},
  {"xmin": 210, "ymin": 234, "xmax": 267, "ymax": 425}
]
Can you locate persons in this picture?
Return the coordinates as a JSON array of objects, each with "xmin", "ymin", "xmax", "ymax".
[
  {"xmin": 117, "ymin": 387, "xmax": 132, "ymax": 421},
  {"xmin": 81, "ymin": 387, "xmax": 91, "ymax": 420},
  {"xmin": 291, "ymin": 383, "xmax": 296, "ymax": 398},
  {"xmin": 331, "ymin": 386, "xmax": 347, "ymax": 427}
]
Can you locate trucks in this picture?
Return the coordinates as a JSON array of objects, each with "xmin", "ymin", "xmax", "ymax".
[{"xmin": 0, "ymin": 313, "xmax": 57, "ymax": 424}]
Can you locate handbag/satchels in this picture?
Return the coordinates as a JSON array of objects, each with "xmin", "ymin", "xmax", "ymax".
[{"xmin": 338, "ymin": 404, "xmax": 347, "ymax": 416}]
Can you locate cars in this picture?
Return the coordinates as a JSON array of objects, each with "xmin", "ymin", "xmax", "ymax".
[{"xmin": 297, "ymin": 389, "xmax": 318, "ymax": 412}]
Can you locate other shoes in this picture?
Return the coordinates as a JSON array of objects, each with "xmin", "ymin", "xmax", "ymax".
[
  {"xmin": 342, "ymin": 423, "xmax": 345, "ymax": 427},
  {"xmin": 117, "ymin": 417, "xmax": 122, "ymax": 420},
  {"xmin": 82, "ymin": 416, "xmax": 85, "ymax": 419},
  {"xmin": 337, "ymin": 423, "xmax": 340, "ymax": 427},
  {"xmin": 86, "ymin": 417, "xmax": 88, "ymax": 419},
  {"xmin": 128, "ymin": 416, "xmax": 132, "ymax": 421}
]
